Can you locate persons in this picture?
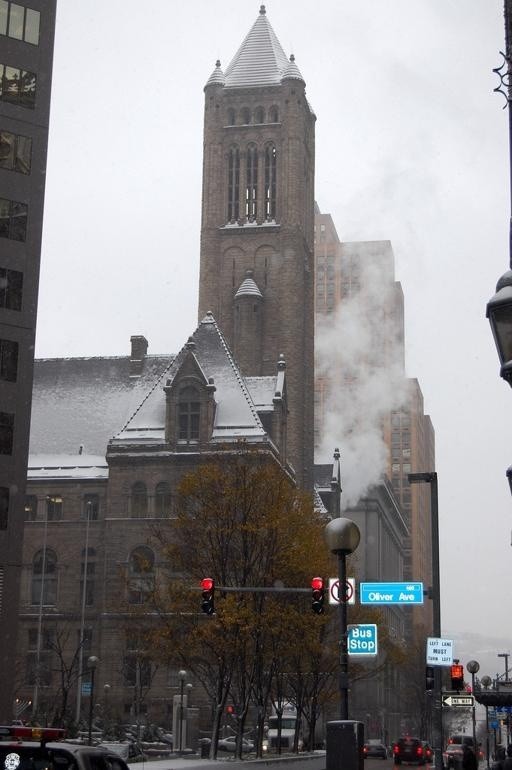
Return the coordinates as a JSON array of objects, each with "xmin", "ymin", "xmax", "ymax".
[{"xmin": 461, "ymin": 744, "xmax": 479, "ymax": 770}]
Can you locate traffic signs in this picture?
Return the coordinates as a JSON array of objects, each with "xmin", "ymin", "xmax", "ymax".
[
  {"xmin": 424, "ymin": 637, "xmax": 455, "ymax": 668},
  {"xmin": 360, "ymin": 582, "xmax": 425, "ymax": 605},
  {"xmin": 440, "ymin": 694, "xmax": 476, "ymax": 709}
]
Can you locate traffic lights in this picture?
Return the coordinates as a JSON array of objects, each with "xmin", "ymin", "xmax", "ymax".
[
  {"xmin": 451, "ymin": 664, "xmax": 465, "ymax": 691},
  {"xmin": 200, "ymin": 578, "xmax": 216, "ymax": 611},
  {"xmin": 223, "ymin": 705, "xmax": 242, "ymax": 715},
  {"xmin": 324, "ymin": 519, "xmax": 367, "ymax": 768},
  {"xmin": 311, "ymin": 577, "xmax": 324, "ymax": 612}
]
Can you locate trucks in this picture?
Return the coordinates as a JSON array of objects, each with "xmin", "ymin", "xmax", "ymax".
[{"xmin": 266, "ymin": 698, "xmax": 325, "ymax": 753}]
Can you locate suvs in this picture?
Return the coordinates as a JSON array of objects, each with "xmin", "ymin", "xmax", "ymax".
[
  {"xmin": 0, "ymin": 726, "xmax": 130, "ymax": 770},
  {"xmin": 393, "ymin": 736, "xmax": 426, "ymax": 766},
  {"xmin": 131, "ymin": 724, "xmax": 173, "ymax": 745}
]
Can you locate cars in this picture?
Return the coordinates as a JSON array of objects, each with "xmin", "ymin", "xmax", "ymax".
[
  {"xmin": 363, "ymin": 738, "xmax": 389, "ymax": 759},
  {"xmin": 422, "ymin": 741, "xmax": 435, "ymax": 762},
  {"xmin": 215, "ymin": 737, "xmax": 255, "ymax": 756},
  {"xmin": 440, "ymin": 734, "xmax": 484, "ymax": 768}
]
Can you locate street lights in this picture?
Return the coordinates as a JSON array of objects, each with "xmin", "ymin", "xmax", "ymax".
[
  {"xmin": 486, "ymin": 268, "xmax": 512, "ymax": 388},
  {"xmin": 498, "ymin": 653, "xmax": 510, "ymax": 748},
  {"xmin": 407, "ymin": 471, "xmax": 444, "ymax": 770},
  {"xmin": 85, "ymin": 655, "xmax": 101, "ymax": 747},
  {"xmin": 467, "ymin": 661, "xmax": 481, "ymax": 750},
  {"xmin": 178, "ymin": 671, "xmax": 187, "ymax": 754},
  {"xmin": 481, "ymin": 675, "xmax": 492, "ymax": 769},
  {"xmin": 103, "ymin": 684, "xmax": 111, "ymax": 709},
  {"xmin": 186, "ymin": 683, "xmax": 193, "ymax": 707}
]
